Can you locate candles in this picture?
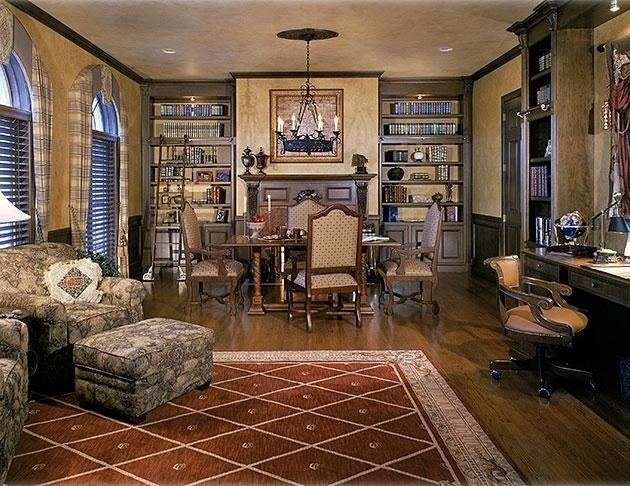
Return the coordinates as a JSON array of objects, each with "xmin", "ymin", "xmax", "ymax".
[{"xmin": 267, "ymin": 195, "xmax": 272, "ymax": 212}]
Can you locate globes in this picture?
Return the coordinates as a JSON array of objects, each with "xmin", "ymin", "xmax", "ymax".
[{"xmin": 552, "ymin": 210, "xmax": 594, "ymax": 257}]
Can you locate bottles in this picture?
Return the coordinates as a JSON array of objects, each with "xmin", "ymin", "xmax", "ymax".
[{"xmin": 175, "ymin": 208, "xmax": 180, "ymax": 223}]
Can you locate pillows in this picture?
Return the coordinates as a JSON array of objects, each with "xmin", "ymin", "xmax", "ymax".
[{"xmin": 43, "ymin": 254, "xmax": 106, "ymax": 304}]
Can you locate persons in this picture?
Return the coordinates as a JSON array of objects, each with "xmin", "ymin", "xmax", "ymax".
[{"xmin": 611, "ymin": 35, "xmax": 630, "ymax": 213}]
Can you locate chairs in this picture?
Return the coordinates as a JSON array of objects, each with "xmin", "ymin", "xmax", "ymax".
[
  {"xmin": 283, "ymin": 203, "xmax": 363, "ymax": 334},
  {"xmin": 177, "ymin": 202, "xmax": 247, "ymax": 316},
  {"xmin": 483, "ymin": 254, "xmax": 603, "ymax": 401},
  {"xmin": 376, "ymin": 198, "xmax": 444, "ymax": 316},
  {"xmin": 284, "ymin": 192, "xmax": 332, "ymax": 275}
]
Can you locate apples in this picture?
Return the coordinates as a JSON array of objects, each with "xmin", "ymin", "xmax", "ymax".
[{"xmin": 246, "ymin": 214, "xmax": 265, "ymax": 223}]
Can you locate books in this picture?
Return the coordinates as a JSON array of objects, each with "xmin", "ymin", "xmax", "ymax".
[
  {"xmin": 383, "ymin": 100, "xmax": 460, "ymax": 222},
  {"xmin": 531, "ymin": 53, "xmax": 551, "ymax": 246},
  {"xmin": 156, "ymin": 104, "xmax": 229, "ymax": 205}
]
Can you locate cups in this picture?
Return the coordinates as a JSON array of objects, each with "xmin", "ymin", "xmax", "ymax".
[
  {"xmin": 293, "ymin": 227, "xmax": 306, "ymax": 238},
  {"xmin": 280, "ymin": 226, "xmax": 292, "ymax": 239}
]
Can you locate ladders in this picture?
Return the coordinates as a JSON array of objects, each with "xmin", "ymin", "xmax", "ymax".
[{"xmin": 151, "ymin": 134, "xmax": 188, "ymax": 279}]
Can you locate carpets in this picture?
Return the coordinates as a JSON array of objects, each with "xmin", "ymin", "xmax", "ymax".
[{"xmin": 7, "ymin": 347, "xmax": 534, "ymax": 486}]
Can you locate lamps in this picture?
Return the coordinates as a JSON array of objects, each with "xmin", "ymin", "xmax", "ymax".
[
  {"xmin": 0, "ymin": 191, "xmax": 32, "ymax": 224},
  {"xmin": 608, "ymin": 0, "xmax": 623, "ymax": 11},
  {"xmin": 591, "ymin": 192, "xmax": 630, "ymax": 263},
  {"xmin": 275, "ymin": 26, "xmax": 341, "ymax": 157}
]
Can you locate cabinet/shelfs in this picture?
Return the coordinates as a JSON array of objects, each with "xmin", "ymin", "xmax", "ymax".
[
  {"xmin": 380, "ymin": 81, "xmax": 475, "ymax": 274},
  {"xmin": 521, "ymin": 33, "xmax": 595, "ymax": 249},
  {"xmin": 149, "ymin": 94, "xmax": 235, "ymax": 262}
]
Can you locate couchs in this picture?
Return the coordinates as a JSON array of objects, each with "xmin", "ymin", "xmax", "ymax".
[
  {"xmin": 0, "ymin": 316, "xmax": 31, "ymax": 485},
  {"xmin": 73, "ymin": 315, "xmax": 215, "ymax": 424},
  {"xmin": 0, "ymin": 241, "xmax": 148, "ymax": 403}
]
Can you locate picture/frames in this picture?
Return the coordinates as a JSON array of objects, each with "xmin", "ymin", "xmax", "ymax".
[{"xmin": 269, "ymin": 87, "xmax": 345, "ymax": 164}]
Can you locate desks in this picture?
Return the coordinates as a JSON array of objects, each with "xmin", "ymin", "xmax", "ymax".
[{"xmin": 515, "ymin": 246, "xmax": 630, "ymax": 366}]
